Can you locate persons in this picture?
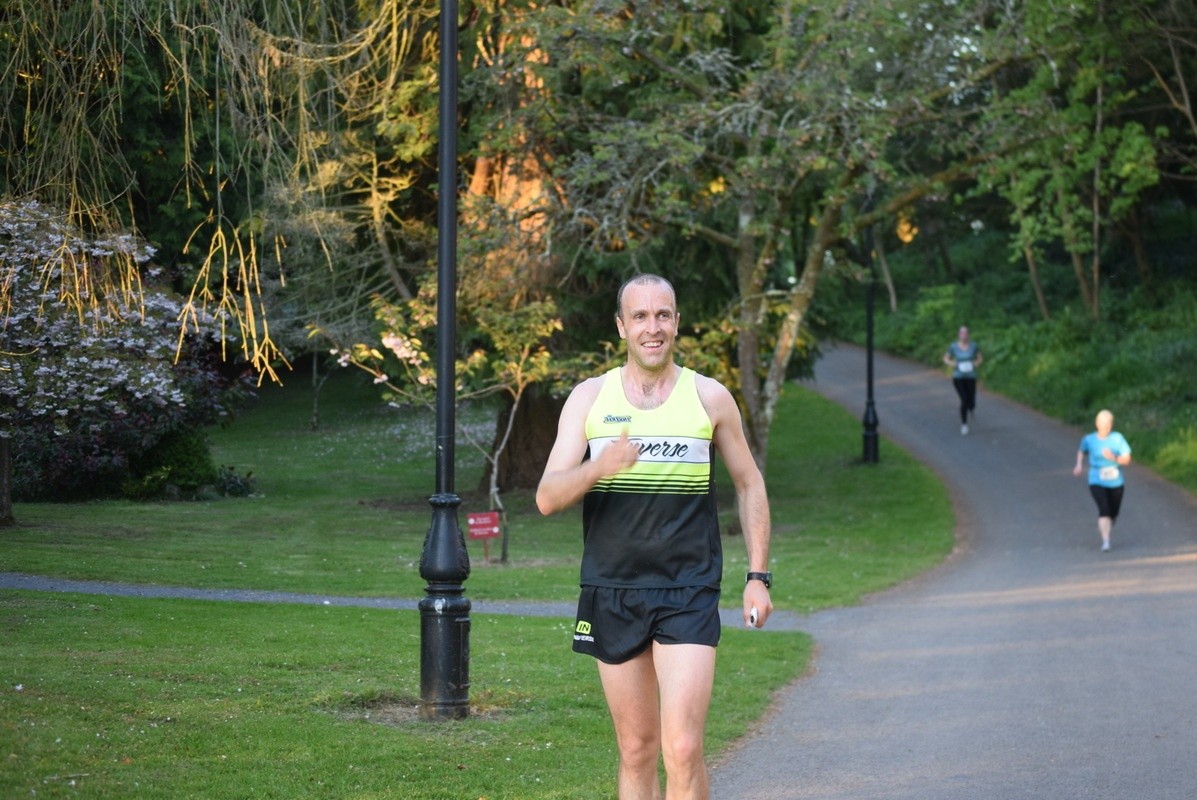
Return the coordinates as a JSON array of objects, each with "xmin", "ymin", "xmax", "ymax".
[
  {"xmin": 943, "ymin": 326, "xmax": 983, "ymax": 435},
  {"xmin": 1073, "ymin": 409, "xmax": 1131, "ymax": 554},
  {"xmin": 536, "ymin": 273, "xmax": 773, "ymax": 800}
]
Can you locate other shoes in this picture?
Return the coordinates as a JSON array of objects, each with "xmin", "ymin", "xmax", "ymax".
[
  {"xmin": 961, "ymin": 424, "xmax": 969, "ymax": 435},
  {"xmin": 1103, "ymin": 540, "xmax": 1111, "ymax": 550}
]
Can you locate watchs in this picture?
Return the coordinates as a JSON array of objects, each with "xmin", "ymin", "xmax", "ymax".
[{"xmin": 744, "ymin": 571, "xmax": 772, "ymax": 588}]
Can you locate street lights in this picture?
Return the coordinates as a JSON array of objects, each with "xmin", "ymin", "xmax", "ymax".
[{"xmin": 849, "ymin": 161, "xmax": 879, "ymax": 461}]
